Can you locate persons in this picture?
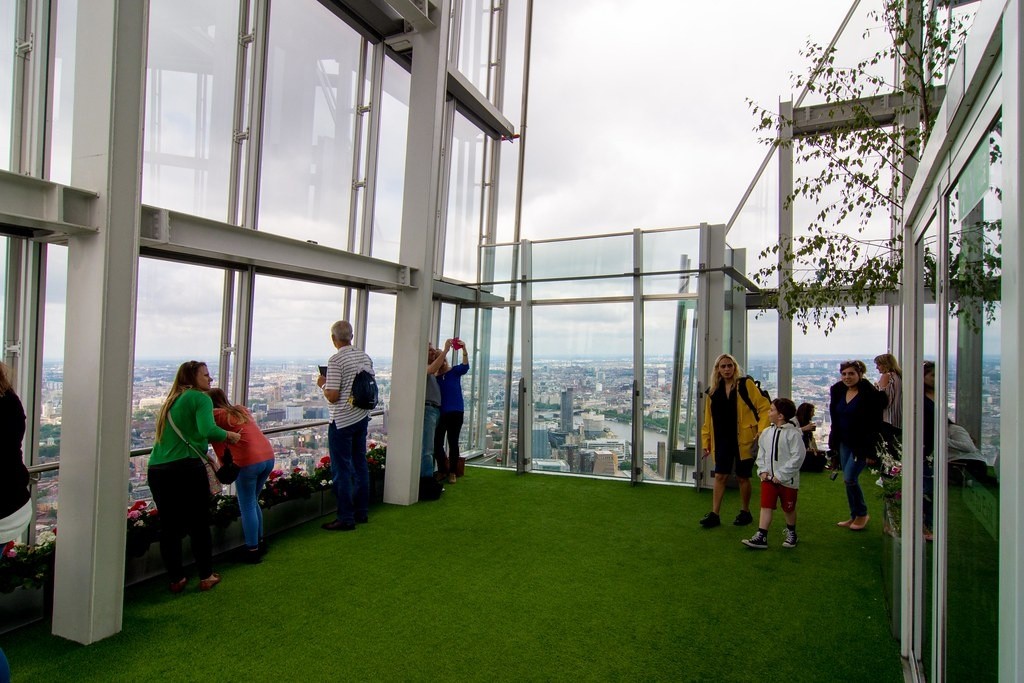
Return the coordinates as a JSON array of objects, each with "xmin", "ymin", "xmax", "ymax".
[
  {"xmin": 203, "ymin": 388, "xmax": 275, "ymax": 563},
  {"xmin": 148, "ymin": 361, "xmax": 242, "ymax": 595},
  {"xmin": 741, "ymin": 398, "xmax": 806, "ymax": 549},
  {"xmin": 434, "ymin": 340, "xmax": 470, "ymax": 484},
  {"xmin": 0, "ymin": 362, "xmax": 32, "ymax": 683},
  {"xmin": 922, "ymin": 361, "xmax": 989, "ymax": 541},
  {"xmin": 789, "ymin": 403, "xmax": 827, "ymax": 472},
  {"xmin": 316, "ymin": 320, "xmax": 376, "ymax": 530},
  {"xmin": 828, "ymin": 354, "xmax": 903, "ymax": 530},
  {"xmin": 700, "ymin": 354, "xmax": 771, "ymax": 525},
  {"xmin": 420, "ymin": 338, "xmax": 453, "ymax": 492}
]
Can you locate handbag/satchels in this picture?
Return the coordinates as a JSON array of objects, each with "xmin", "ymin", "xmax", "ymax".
[{"xmin": 204, "ymin": 457, "xmax": 223, "ymax": 495}]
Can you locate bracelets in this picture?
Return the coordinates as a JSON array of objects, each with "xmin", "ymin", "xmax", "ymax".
[
  {"xmin": 463, "ymin": 353, "xmax": 468, "ymax": 356},
  {"xmin": 322, "ymin": 383, "xmax": 326, "ymax": 388}
]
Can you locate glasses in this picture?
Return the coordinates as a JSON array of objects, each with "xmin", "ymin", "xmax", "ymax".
[{"xmin": 840, "ymin": 361, "xmax": 857, "ymax": 366}]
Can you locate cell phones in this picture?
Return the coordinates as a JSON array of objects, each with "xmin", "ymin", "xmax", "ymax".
[{"xmin": 452, "ymin": 337, "xmax": 463, "ymax": 351}]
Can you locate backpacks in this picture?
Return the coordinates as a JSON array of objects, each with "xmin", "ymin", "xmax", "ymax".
[
  {"xmin": 739, "ymin": 375, "xmax": 773, "ymax": 422},
  {"xmin": 347, "ymin": 370, "xmax": 379, "ymax": 410}
]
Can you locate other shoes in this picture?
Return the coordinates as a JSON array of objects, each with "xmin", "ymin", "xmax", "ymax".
[
  {"xmin": 172, "ymin": 578, "xmax": 188, "ymax": 592},
  {"xmin": 200, "ymin": 573, "xmax": 221, "ymax": 590},
  {"xmin": 449, "ymin": 474, "xmax": 457, "ymax": 484},
  {"xmin": 923, "ymin": 534, "xmax": 934, "ymax": 540},
  {"xmin": 249, "ymin": 547, "xmax": 262, "ymax": 564},
  {"xmin": 259, "ymin": 541, "xmax": 268, "ymax": 553},
  {"xmin": 837, "ymin": 521, "xmax": 853, "ymax": 527},
  {"xmin": 850, "ymin": 515, "xmax": 870, "ymax": 530}
]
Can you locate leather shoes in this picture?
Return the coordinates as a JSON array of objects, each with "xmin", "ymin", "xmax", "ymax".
[{"xmin": 321, "ymin": 517, "xmax": 353, "ymax": 532}]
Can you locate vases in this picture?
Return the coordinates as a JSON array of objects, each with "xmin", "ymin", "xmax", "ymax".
[
  {"xmin": 0, "ymin": 585, "xmax": 52, "ymax": 635},
  {"xmin": 880, "ymin": 499, "xmax": 901, "ymax": 641},
  {"xmin": 261, "ymin": 472, "xmax": 384, "ymax": 538},
  {"xmin": 125, "ymin": 515, "xmax": 246, "ymax": 587}
]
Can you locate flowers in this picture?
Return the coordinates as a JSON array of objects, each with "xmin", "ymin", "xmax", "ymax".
[
  {"xmin": 127, "ymin": 501, "xmax": 160, "ymax": 543},
  {"xmin": 258, "ymin": 455, "xmax": 333, "ymax": 509},
  {"xmin": 0, "ymin": 528, "xmax": 56, "ymax": 594},
  {"xmin": 210, "ymin": 494, "xmax": 241, "ymax": 529},
  {"xmin": 365, "ymin": 443, "xmax": 385, "ymax": 474},
  {"xmin": 866, "ymin": 431, "xmax": 901, "ymax": 503}
]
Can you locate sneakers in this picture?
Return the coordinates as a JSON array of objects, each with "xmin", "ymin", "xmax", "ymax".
[
  {"xmin": 733, "ymin": 509, "xmax": 753, "ymax": 526},
  {"xmin": 742, "ymin": 530, "xmax": 768, "ymax": 549},
  {"xmin": 700, "ymin": 511, "xmax": 720, "ymax": 526},
  {"xmin": 783, "ymin": 530, "xmax": 800, "ymax": 548}
]
